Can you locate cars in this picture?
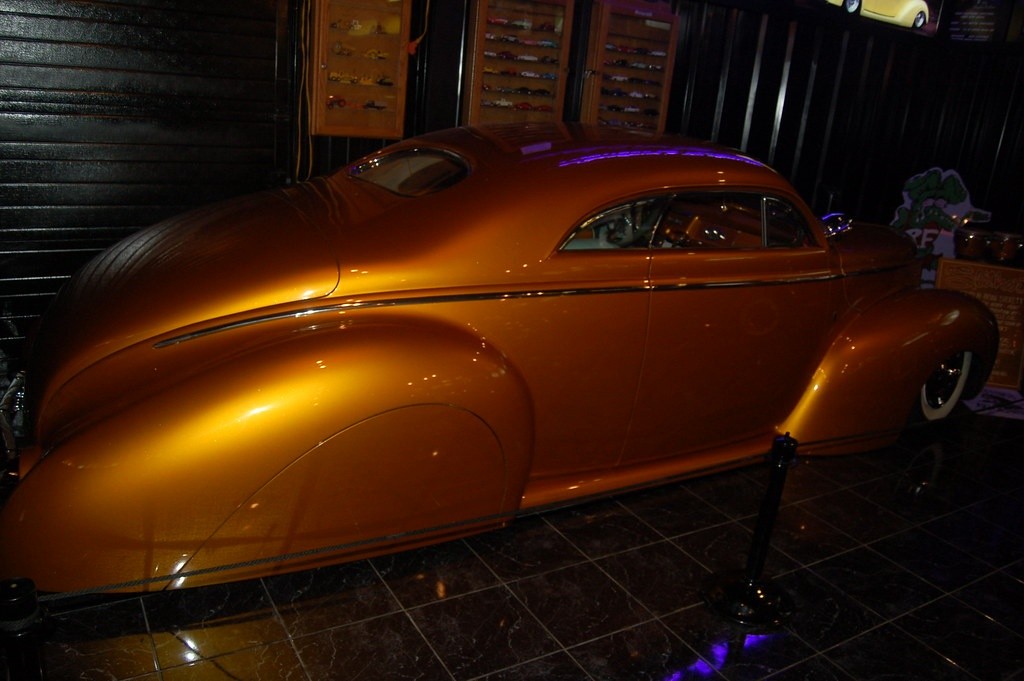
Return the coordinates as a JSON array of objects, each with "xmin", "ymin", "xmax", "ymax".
[{"xmin": 0, "ymin": 121, "xmax": 999, "ymax": 594}]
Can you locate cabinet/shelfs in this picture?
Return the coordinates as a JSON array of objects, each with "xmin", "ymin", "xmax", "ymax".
[
  {"xmin": 579, "ymin": 1, "xmax": 682, "ymax": 133},
  {"xmin": 462, "ymin": 0, "xmax": 575, "ymax": 127},
  {"xmin": 309, "ymin": 0, "xmax": 412, "ymax": 140}
]
